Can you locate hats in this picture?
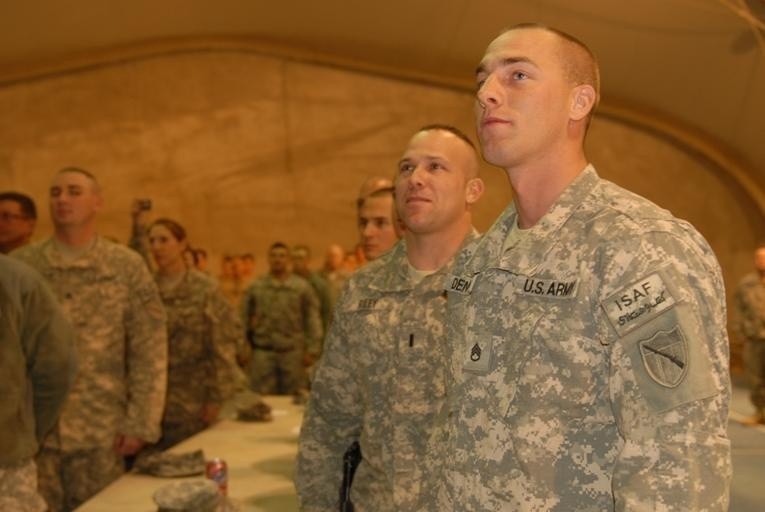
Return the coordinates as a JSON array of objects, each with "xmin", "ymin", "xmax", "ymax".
[
  {"xmin": 154, "ymin": 478, "xmax": 240, "ymax": 512},
  {"xmin": 234, "ymin": 400, "xmax": 274, "ymax": 422},
  {"xmin": 134, "ymin": 446, "xmax": 209, "ymax": 476}
]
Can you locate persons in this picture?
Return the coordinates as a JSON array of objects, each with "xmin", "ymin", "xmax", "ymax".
[
  {"xmin": 146, "ymin": 217, "xmax": 240, "ymax": 453},
  {"xmin": 181, "ymin": 247, "xmax": 212, "ymax": 275},
  {"xmin": 125, "ymin": 197, "xmax": 157, "ymax": 276},
  {"xmin": 416, "ymin": 23, "xmax": 730, "ymax": 511},
  {"xmin": 0, "ymin": 191, "xmax": 37, "ymax": 255},
  {"xmin": 220, "ymin": 243, "xmax": 359, "ymax": 394},
  {"xmin": 356, "ymin": 163, "xmax": 407, "ymax": 262},
  {"xmin": 0, "ymin": 256, "xmax": 79, "ymax": 512},
  {"xmin": 293, "ymin": 125, "xmax": 487, "ymax": 512},
  {"xmin": 730, "ymin": 245, "xmax": 765, "ymax": 429},
  {"xmin": 9, "ymin": 167, "xmax": 168, "ymax": 510}
]
